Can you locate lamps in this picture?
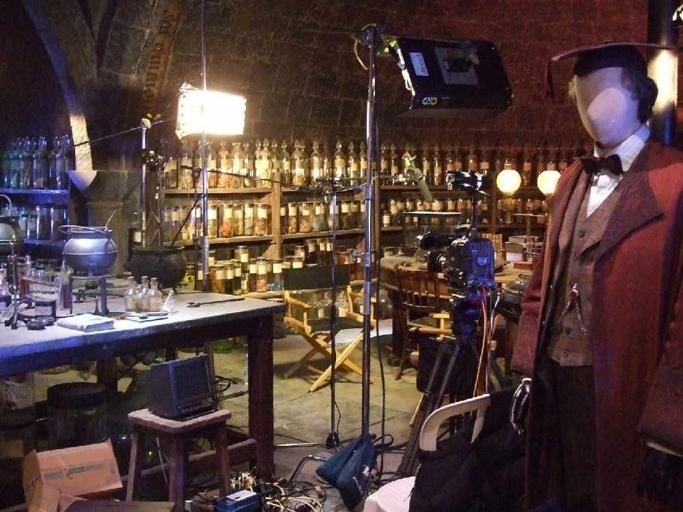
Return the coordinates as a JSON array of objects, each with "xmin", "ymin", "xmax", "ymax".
[{"xmin": 382, "ymin": 34, "xmax": 514, "ymax": 111}]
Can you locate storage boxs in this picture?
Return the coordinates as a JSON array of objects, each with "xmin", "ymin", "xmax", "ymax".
[{"xmin": 22, "ymin": 436, "xmax": 123, "ymax": 511}]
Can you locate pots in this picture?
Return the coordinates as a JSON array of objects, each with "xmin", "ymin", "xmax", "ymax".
[
  {"xmin": 131, "ymin": 246, "xmax": 188, "ymax": 295},
  {"xmin": 58, "ymin": 224, "xmax": 119, "ymax": 275},
  {"xmin": 0, "ymin": 194, "xmax": 24, "ymax": 259}
]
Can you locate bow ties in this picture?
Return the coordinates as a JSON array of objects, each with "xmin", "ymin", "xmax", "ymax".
[{"xmin": 580, "ymin": 155, "xmax": 622, "ymax": 173}]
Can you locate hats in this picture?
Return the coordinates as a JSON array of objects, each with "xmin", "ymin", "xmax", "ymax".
[{"xmin": 550, "ymin": 42, "xmax": 675, "ymax": 77}]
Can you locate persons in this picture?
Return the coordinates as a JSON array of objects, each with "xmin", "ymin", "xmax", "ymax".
[{"xmin": 500, "ymin": 41, "xmax": 682, "ymax": 512}]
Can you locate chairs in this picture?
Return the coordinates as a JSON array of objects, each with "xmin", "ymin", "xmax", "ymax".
[
  {"xmin": 362, "ymin": 388, "xmax": 524, "ymax": 512},
  {"xmin": 281, "ymin": 265, "xmax": 377, "ymax": 393},
  {"xmin": 395, "ymin": 267, "xmax": 452, "ymax": 381}
]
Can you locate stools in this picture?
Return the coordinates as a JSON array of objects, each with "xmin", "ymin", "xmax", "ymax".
[{"xmin": 125, "ymin": 407, "xmax": 233, "ymax": 512}]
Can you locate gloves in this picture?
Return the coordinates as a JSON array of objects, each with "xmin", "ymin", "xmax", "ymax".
[
  {"xmin": 637, "ymin": 445, "xmax": 682, "ymax": 505},
  {"xmin": 509, "ymin": 380, "xmax": 532, "ymax": 438}
]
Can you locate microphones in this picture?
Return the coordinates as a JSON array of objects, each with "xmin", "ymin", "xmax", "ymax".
[{"xmin": 409, "ymin": 161, "xmax": 434, "ymax": 202}]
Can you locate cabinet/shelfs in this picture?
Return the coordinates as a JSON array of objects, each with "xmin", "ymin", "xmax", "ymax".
[
  {"xmin": 146, "ymin": 159, "xmax": 551, "ymax": 286},
  {"xmin": 0, "ymin": 158, "xmax": 87, "ymax": 265}
]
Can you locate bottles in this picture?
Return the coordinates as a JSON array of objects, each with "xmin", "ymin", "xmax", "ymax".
[
  {"xmin": 157, "ymin": 133, "xmax": 518, "ymax": 191},
  {"xmin": 181, "ymin": 237, "xmax": 405, "ymax": 296},
  {"xmin": 126, "ymin": 275, "xmax": 164, "ymax": 313},
  {"xmin": 22, "ymin": 266, "xmax": 71, "ymax": 310},
  {"xmin": 310, "ymin": 289, "xmax": 373, "ymax": 320},
  {"xmin": 3, "ymin": 135, "xmax": 74, "ymax": 189},
  {"xmin": 14, "ymin": 203, "xmax": 69, "ymax": 242},
  {"xmin": 161, "ymin": 198, "xmax": 550, "ymax": 239}
]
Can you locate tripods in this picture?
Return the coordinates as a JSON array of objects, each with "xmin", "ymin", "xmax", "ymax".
[
  {"xmin": 395, "ymin": 321, "xmax": 503, "ymax": 477},
  {"xmin": 273, "ymin": 168, "xmax": 413, "ymax": 458}
]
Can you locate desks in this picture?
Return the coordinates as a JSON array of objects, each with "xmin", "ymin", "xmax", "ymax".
[
  {"xmin": 0, "ymin": 290, "xmax": 289, "ymax": 507},
  {"xmin": 377, "ymin": 255, "xmax": 554, "ymax": 365}
]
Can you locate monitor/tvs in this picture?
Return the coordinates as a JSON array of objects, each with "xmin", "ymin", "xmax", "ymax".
[{"xmin": 149, "ymin": 355, "xmax": 219, "ymax": 421}]
